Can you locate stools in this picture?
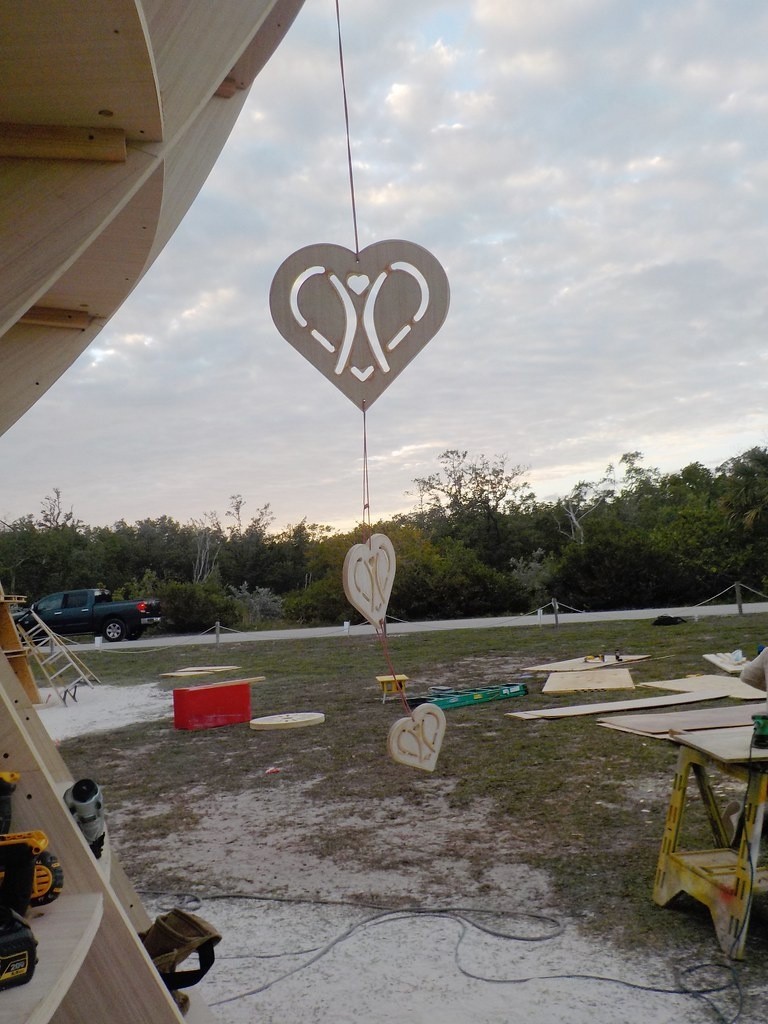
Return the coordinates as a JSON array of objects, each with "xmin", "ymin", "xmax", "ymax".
[{"xmin": 376, "ymin": 674, "xmax": 409, "ymax": 704}]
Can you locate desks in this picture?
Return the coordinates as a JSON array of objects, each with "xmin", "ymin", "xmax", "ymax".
[{"xmin": 651, "ymin": 727, "xmax": 768, "ymax": 957}]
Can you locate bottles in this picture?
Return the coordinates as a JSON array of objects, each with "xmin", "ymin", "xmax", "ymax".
[
  {"xmin": 614, "ymin": 649, "xmax": 620, "ymax": 660},
  {"xmin": 598, "ymin": 654, "xmax": 604, "ymax": 661}
]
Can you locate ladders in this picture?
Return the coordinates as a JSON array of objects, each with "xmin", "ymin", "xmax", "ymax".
[
  {"xmin": 406, "ymin": 682, "xmax": 528, "ymax": 711},
  {"xmin": 15, "ymin": 608, "xmax": 101, "ymax": 708}
]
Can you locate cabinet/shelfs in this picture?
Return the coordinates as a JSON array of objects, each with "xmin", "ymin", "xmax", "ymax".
[{"xmin": 0, "ymin": 583, "xmax": 187, "ymax": 1024}]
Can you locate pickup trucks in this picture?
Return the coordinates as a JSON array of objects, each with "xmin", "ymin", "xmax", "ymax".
[{"xmin": 11, "ymin": 588, "xmax": 163, "ymax": 641}]
